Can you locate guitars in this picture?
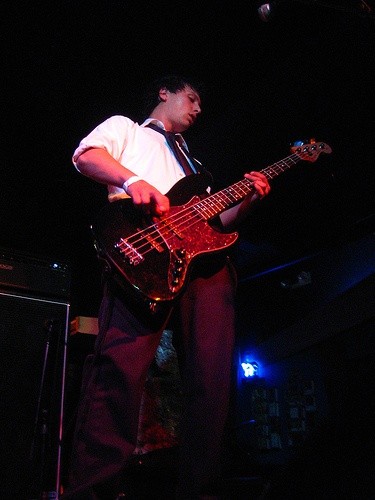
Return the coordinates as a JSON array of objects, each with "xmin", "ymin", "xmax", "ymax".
[{"xmin": 89, "ymin": 138, "xmax": 333, "ymax": 312}]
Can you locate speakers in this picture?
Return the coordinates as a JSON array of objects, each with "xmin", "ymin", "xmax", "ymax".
[{"xmin": 0, "ymin": 289, "xmax": 70, "ymax": 500}]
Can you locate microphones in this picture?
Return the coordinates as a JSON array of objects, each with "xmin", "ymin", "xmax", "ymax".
[
  {"xmin": 258, "ymin": 0, "xmax": 281, "ymax": 22},
  {"xmin": 42, "ymin": 318, "xmax": 56, "ymax": 331}
]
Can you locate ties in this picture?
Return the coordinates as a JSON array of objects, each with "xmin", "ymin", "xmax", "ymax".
[{"xmin": 144, "ymin": 122, "xmax": 194, "ymax": 176}]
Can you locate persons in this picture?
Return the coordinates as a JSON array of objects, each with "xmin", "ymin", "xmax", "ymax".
[{"xmin": 64, "ymin": 76, "xmax": 272, "ymax": 500}]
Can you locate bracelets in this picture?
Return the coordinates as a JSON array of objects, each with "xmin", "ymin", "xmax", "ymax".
[{"xmin": 122, "ymin": 176, "xmax": 144, "ymax": 190}]
